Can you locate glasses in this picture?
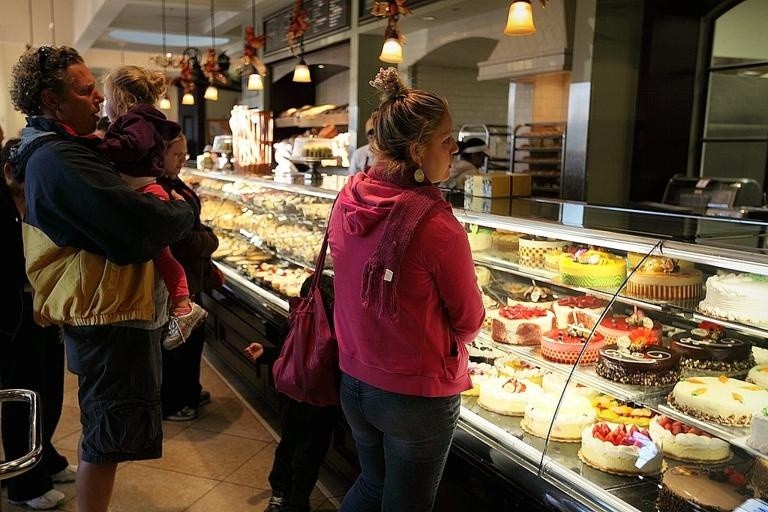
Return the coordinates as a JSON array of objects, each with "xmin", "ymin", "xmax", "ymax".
[{"xmin": 165, "ymin": 152, "xmax": 190, "ymax": 161}]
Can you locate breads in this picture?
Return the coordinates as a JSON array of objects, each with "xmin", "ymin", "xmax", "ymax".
[{"xmin": 177, "ymin": 172, "xmax": 335, "ymax": 299}]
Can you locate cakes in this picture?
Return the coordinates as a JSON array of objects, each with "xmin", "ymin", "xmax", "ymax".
[
  {"xmin": 460, "ymin": 221, "xmax": 766, "ymax": 325},
  {"xmin": 217, "ymin": 143, "xmax": 232, "ymax": 150},
  {"xmin": 657, "ymin": 464, "xmax": 756, "ymax": 512},
  {"xmin": 470, "ymin": 263, "xmax": 767, "ymax": 453},
  {"xmin": 302, "ymin": 147, "xmax": 332, "ymax": 157},
  {"xmin": 465, "ymin": 338, "xmax": 734, "ymax": 479}
]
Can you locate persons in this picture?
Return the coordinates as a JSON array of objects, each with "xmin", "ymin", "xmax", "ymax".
[
  {"xmin": 240, "ymin": 272, "xmax": 341, "ymax": 510},
  {"xmin": 326, "ymin": 68, "xmax": 486, "ymax": 512},
  {"xmin": 348, "ymin": 118, "xmax": 381, "ymax": 180},
  {"xmin": 441, "ymin": 134, "xmax": 493, "ymax": 197},
  {"xmin": 1, "ymin": 44, "xmax": 228, "ymax": 511}
]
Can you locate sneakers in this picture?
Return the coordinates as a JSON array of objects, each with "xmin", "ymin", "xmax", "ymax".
[
  {"xmin": 41, "ymin": 461, "xmax": 84, "ymax": 484},
  {"xmin": 185, "ymin": 390, "xmax": 211, "ymax": 408},
  {"xmin": 162, "ymin": 301, "xmax": 213, "ymax": 353},
  {"xmin": 7, "ymin": 485, "xmax": 69, "ymax": 511},
  {"xmin": 160, "ymin": 407, "xmax": 204, "ymax": 423}
]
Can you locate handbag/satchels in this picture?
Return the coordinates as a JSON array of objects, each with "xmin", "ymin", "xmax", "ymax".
[{"xmin": 268, "ymin": 281, "xmax": 344, "ymax": 411}]
